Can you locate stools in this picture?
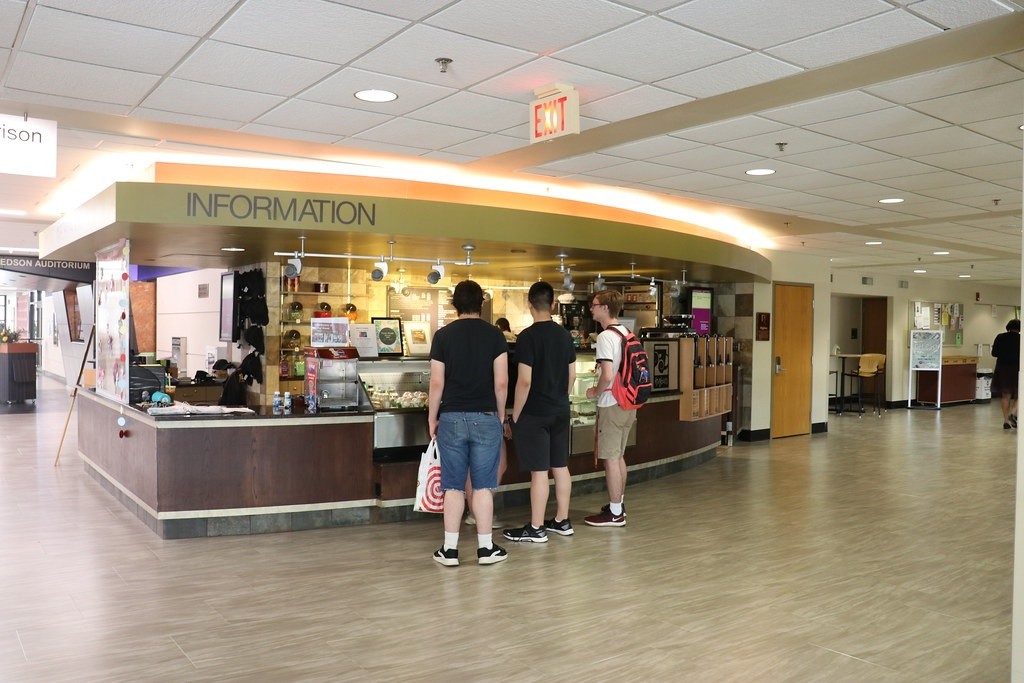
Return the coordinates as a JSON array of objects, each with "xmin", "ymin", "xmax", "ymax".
[{"xmin": 849, "ymin": 354, "xmax": 882, "ymax": 418}]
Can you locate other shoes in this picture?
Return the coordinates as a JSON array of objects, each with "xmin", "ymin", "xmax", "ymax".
[{"xmin": 464, "ymin": 511, "xmax": 505, "ymax": 528}]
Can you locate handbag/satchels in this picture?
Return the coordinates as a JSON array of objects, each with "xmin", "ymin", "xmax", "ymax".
[{"xmin": 413, "ymin": 437, "xmax": 445, "ymax": 513}]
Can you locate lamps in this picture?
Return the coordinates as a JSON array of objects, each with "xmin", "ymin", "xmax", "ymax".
[{"xmin": 285, "ymin": 245, "xmax": 681, "ymax": 299}]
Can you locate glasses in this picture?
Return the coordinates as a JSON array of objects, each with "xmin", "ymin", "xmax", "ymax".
[{"xmin": 589, "ymin": 302, "xmax": 609, "ymax": 309}]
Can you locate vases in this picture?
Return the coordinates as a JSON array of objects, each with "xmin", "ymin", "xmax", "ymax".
[{"xmin": 5, "ymin": 335, "xmax": 14, "ymax": 343}]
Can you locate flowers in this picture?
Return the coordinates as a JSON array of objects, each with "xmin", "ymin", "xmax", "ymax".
[{"xmin": 0, "ymin": 323, "xmax": 24, "ymax": 342}]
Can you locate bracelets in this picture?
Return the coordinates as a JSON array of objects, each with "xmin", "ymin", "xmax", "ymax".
[{"xmin": 503, "ymin": 420, "xmax": 510, "ymax": 424}]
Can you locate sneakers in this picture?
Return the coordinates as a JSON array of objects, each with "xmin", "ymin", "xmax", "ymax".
[
  {"xmin": 584, "ymin": 503, "xmax": 627, "ymax": 526},
  {"xmin": 477, "ymin": 541, "xmax": 508, "ymax": 565},
  {"xmin": 1003, "ymin": 414, "xmax": 1017, "ymax": 429},
  {"xmin": 433, "ymin": 544, "xmax": 459, "ymax": 567},
  {"xmin": 544, "ymin": 517, "xmax": 575, "ymax": 536},
  {"xmin": 502, "ymin": 521, "xmax": 549, "ymax": 543}
]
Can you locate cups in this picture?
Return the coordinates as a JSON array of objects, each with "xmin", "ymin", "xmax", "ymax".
[
  {"xmin": 281, "ymin": 330, "xmax": 301, "ymax": 347},
  {"xmin": 166, "ymin": 386, "xmax": 176, "ymax": 406},
  {"xmin": 315, "ymin": 283, "xmax": 329, "ymax": 293},
  {"xmin": 284, "ymin": 275, "xmax": 300, "ymax": 292},
  {"xmin": 283, "ymin": 302, "xmax": 357, "ymax": 324}
]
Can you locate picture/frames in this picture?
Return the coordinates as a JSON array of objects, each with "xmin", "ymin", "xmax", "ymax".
[
  {"xmin": 373, "ymin": 316, "xmax": 401, "ymax": 354},
  {"xmin": 755, "ymin": 312, "xmax": 770, "ymax": 341},
  {"xmin": 401, "ymin": 322, "xmax": 433, "ymax": 355}
]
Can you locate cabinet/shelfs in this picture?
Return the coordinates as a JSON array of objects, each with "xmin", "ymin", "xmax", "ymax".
[
  {"xmin": 172, "ymin": 384, "xmax": 227, "ymax": 405},
  {"xmin": 279, "ymin": 290, "xmax": 373, "ymax": 381}
]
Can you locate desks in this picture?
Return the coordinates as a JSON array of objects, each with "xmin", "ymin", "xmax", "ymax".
[
  {"xmin": 1, "ymin": 342, "xmax": 40, "ymax": 406},
  {"xmin": 916, "ymin": 356, "xmax": 979, "ymax": 406},
  {"xmin": 830, "ymin": 353, "xmax": 885, "ymax": 415}
]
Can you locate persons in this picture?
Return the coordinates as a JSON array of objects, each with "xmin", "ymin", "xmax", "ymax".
[
  {"xmin": 583, "ymin": 290, "xmax": 641, "ymax": 527},
  {"xmin": 503, "ymin": 281, "xmax": 577, "ymax": 540},
  {"xmin": 427, "ymin": 279, "xmax": 508, "ymax": 568},
  {"xmin": 465, "ymin": 404, "xmax": 512, "ymax": 528},
  {"xmin": 494, "ymin": 317, "xmax": 511, "ymax": 339},
  {"xmin": 990, "ymin": 319, "xmax": 1020, "ymax": 429}
]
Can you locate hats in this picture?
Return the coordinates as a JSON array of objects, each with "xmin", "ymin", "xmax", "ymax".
[
  {"xmin": 212, "ymin": 359, "xmax": 234, "ymax": 370},
  {"xmin": 237, "ymin": 270, "xmax": 269, "ymax": 385},
  {"xmin": 191, "ymin": 371, "xmax": 216, "ymax": 381}
]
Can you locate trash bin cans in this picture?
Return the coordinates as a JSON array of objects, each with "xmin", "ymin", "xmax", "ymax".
[{"xmin": 971, "ymin": 368, "xmax": 994, "ymax": 403}]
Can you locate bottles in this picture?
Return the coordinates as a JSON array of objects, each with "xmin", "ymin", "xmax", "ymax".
[
  {"xmin": 284, "ymin": 392, "xmax": 292, "ymax": 414},
  {"xmin": 363, "ymin": 381, "xmax": 390, "ymax": 409},
  {"xmin": 273, "ymin": 391, "xmax": 282, "ymax": 414},
  {"xmin": 280, "ymin": 354, "xmax": 305, "ymax": 376}
]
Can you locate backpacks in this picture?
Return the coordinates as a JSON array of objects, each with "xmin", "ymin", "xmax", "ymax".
[{"xmin": 604, "ymin": 324, "xmax": 653, "ymax": 411}]
